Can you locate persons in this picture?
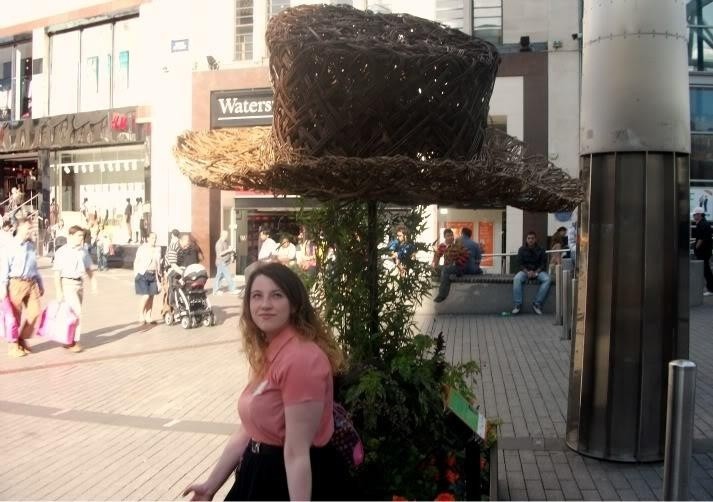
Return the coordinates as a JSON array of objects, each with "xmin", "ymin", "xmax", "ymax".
[
  {"xmin": 123, "ymin": 228, "xmax": 235, "ymax": 326},
  {"xmin": 182, "ymin": 263, "xmax": 353, "ymax": 501},
  {"xmin": 693, "ymin": 206, "xmax": 713, "ymax": 296},
  {"xmin": 425, "ymin": 227, "xmax": 483, "ymax": 303},
  {"xmin": 1, "ymin": 187, "xmax": 115, "ymax": 356},
  {"xmin": 258, "ymin": 221, "xmax": 416, "ymax": 315},
  {"xmin": 509, "ymin": 226, "xmax": 569, "ymax": 315},
  {"xmin": 122, "ymin": 196, "xmax": 148, "ymax": 243}
]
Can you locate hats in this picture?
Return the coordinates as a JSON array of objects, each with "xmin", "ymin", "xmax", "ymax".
[{"xmin": 688, "ymin": 206, "xmax": 704, "ymax": 217}]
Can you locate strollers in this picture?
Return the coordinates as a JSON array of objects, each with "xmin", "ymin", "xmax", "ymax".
[{"xmin": 160, "ymin": 262, "xmax": 218, "ymax": 332}]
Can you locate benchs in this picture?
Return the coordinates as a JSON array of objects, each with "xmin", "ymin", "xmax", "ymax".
[{"xmin": 432, "ymin": 248, "xmax": 570, "ymax": 316}]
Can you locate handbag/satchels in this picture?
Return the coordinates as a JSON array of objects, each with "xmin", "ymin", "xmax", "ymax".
[
  {"xmin": 329, "ymin": 398, "xmax": 366, "ymax": 474},
  {"xmin": 34, "ymin": 298, "xmax": 79, "ymax": 346}
]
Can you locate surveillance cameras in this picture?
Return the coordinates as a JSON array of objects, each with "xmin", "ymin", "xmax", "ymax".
[{"xmin": 571, "ymin": 33, "xmax": 581, "ymax": 41}]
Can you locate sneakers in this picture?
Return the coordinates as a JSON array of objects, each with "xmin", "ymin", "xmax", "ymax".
[
  {"xmin": 511, "ymin": 303, "xmax": 520, "ymax": 314},
  {"xmin": 138, "ymin": 315, "xmax": 157, "ymax": 324},
  {"xmin": 529, "ymin": 300, "xmax": 543, "ymax": 315},
  {"xmin": 432, "ymin": 295, "xmax": 446, "ymax": 303},
  {"xmin": 702, "ymin": 290, "xmax": 713, "ymax": 297},
  {"xmin": 6, "ymin": 341, "xmax": 32, "ymax": 358},
  {"xmin": 62, "ymin": 340, "xmax": 83, "ymax": 353}
]
